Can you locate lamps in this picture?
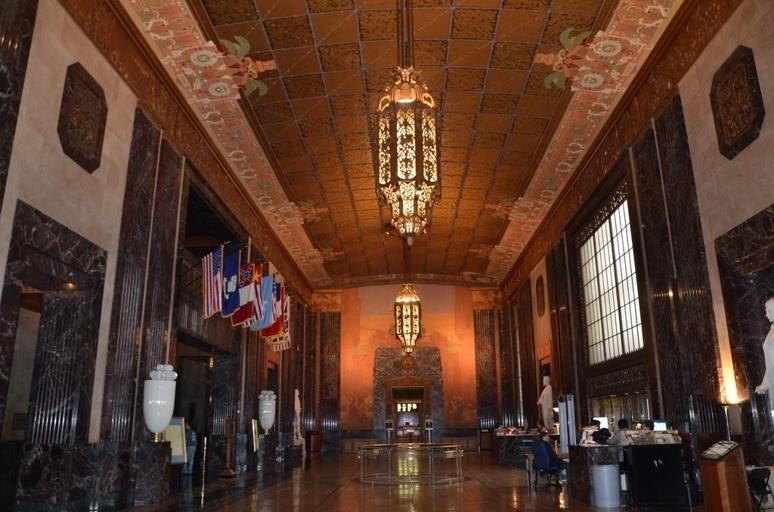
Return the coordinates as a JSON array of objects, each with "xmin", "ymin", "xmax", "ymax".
[
  {"xmin": 371, "ymin": 0, "xmax": 442, "ymax": 353},
  {"xmin": 257, "ymin": 388, "xmax": 277, "ymax": 435},
  {"xmin": 142, "ymin": 363, "xmax": 179, "ymax": 443}
]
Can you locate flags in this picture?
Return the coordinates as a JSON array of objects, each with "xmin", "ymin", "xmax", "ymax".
[
  {"xmin": 201, "ymin": 245, "xmax": 223, "ymax": 320},
  {"xmin": 221, "ymin": 249, "xmax": 241, "ymax": 319},
  {"xmin": 230, "ymin": 260, "xmax": 292, "ymax": 352}
]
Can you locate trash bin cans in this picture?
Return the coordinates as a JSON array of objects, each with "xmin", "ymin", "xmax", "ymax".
[
  {"xmin": 306, "ymin": 431, "xmax": 320, "ymax": 451},
  {"xmin": 587, "ymin": 446, "xmax": 619, "ymax": 507}
]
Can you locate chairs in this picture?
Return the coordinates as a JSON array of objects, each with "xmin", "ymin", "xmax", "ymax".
[
  {"xmin": 532, "ymin": 440, "xmax": 568, "ymax": 490},
  {"xmin": 747, "ymin": 467, "xmax": 774, "ymax": 512}
]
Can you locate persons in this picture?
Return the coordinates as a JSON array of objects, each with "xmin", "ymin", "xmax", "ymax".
[
  {"xmin": 534, "ymin": 432, "xmax": 570, "ymax": 490},
  {"xmin": 536, "ymin": 375, "xmax": 554, "ymax": 430},
  {"xmin": 753, "ymin": 297, "xmax": 774, "ymax": 411},
  {"xmin": 176, "ymin": 474, "xmax": 193, "ymax": 512},
  {"xmin": 181, "ymin": 420, "xmax": 198, "ymax": 475},
  {"xmin": 641, "ymin": 420, "xmax": 655, "ymax": 431},
  {"xmin": 515, "ymin": 420, "xmax": 558, "ymax": 445},
  {"xmin": 617, "ymin": 418, "xmax": 629, "ymax": 431}
]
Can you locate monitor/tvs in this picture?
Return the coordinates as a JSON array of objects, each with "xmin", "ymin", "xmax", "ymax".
[
  {"xmin": 592, "ymin": 416, "xmax": 609, "ymax": 429},
  {"xmin": 653, "ymin": 420, "xmax": 667, "ymax": 432}
]
